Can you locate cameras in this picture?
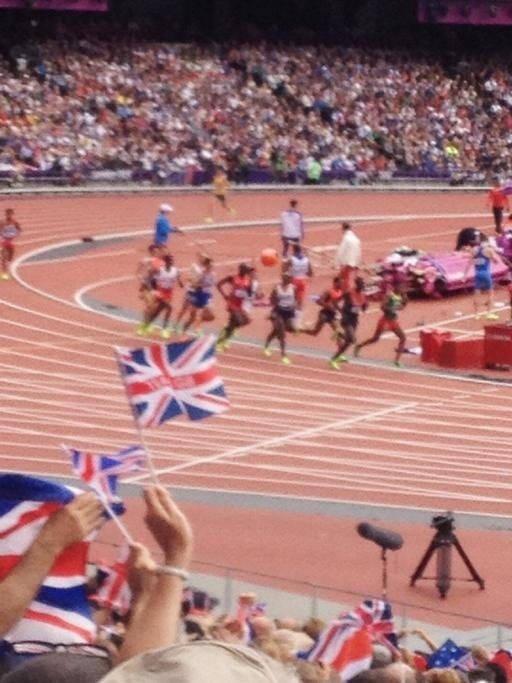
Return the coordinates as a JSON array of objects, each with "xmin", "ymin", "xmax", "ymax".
[{"xmin": 432, "ymin": 516, "xmax": 456, "ymax": 532}]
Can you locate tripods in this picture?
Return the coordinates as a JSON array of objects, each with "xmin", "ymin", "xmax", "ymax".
[{"xmin": 411, "ymin": 533, "xmax": 485, "ymax": 599}]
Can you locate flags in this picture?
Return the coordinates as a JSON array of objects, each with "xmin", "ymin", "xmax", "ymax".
[
  {"xmin": 114, "ymin": 334, "xmax": 230, "ymax": 430},
  {"xmin": 1, "ymin": 445, "xmax": 152, "ymax": 653}
]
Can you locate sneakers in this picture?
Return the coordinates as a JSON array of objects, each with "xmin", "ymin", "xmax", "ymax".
[
  {"xmin": 332, "ymin": 327, "xmax": 360, "ymax": 370},
  {"xmin": 395, "ymin": 361, "xmax": 403, "ymax": 368},
  {"xmin": 474, "ymin": 312, "xmax": 499, "ymax": 321},
  {"xmin": 265, "ymin": 325, "xmax": 301, "ymax": 365},
  {"xmin": 136, "ymin": 321, "xmax": 240, "ymax": 353}
]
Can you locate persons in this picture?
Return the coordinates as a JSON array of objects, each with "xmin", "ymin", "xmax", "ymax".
[
  {"xmin": 1, "ymin": 484, "xmax": 512, "ymax": 683},
  {"xmin": 135, "ymin": 167, "xmax": 409, "ymax": 369},
  {"xmin": 463, "ymin": 179, "xmax": 512, "ymax": 319},
  {"xmin": 2, "ymin": 208, "xmax": 19, "ymax": 280},
  {"xmin": 1, "ymin": 35, "xmax": 511, "ymax": 186}
]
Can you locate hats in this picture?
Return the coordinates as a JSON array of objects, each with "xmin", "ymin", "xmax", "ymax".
[{"xmin": 160, "ymin": 204, "xmax": 174, "ymax": 212}]
